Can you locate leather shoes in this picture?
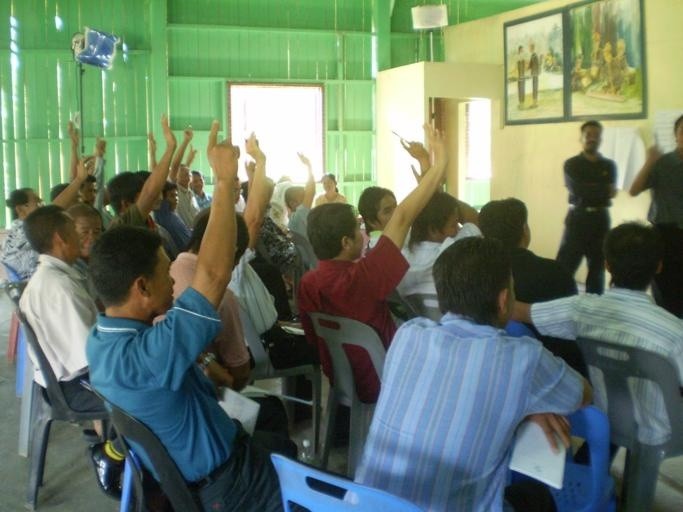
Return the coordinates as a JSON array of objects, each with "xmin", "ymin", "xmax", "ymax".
[{"xmin": 88, "ymin": 442, "xmax": 121, "ymax": 500}]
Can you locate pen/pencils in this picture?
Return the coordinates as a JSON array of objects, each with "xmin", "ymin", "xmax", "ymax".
[{"xmin": 392, "ymin": 131, "xmax": 411, "ymax": 145}]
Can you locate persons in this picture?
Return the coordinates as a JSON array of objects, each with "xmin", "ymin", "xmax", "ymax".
[
  {"xmin": 556, "ymin": 120, "xmax": 619, "ymax": 294},
  {"xmin": 147, "ymin": 113, "xmax": 484, "ymax": 459},
  {"xmin": 344, "ymin": 234, "xmax": 592, "ymax": 512},
  {"xmin": 480, "ymin": 197, "xmax": 591, "ymax": 377},
  {"xmin": 629, "ymin": 118, "xmax": 681, "ymax": 318},
  {"xmin": 0, "ymin": 114, "xmax": 178, "ymax": 500},
  {"xmin": 84, "ymin": 116, "xmax": 355, "ymax": 512},
  {"xmin": 509, "ymin": 222, "xmax": 681, "ymax": 470}
]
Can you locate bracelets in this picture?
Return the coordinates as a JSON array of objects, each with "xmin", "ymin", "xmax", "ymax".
[{"xmin": 198, "ymin": 353, "xmax": 216, "ymax": 371}]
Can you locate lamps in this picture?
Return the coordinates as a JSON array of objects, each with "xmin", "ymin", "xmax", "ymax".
[{"xmin": 412, "ymin": 4, "xmax": 448, "ymax": 129}]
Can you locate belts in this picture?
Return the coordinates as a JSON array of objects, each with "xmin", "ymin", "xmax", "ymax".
[
  {"xmin": 190, "ymin": 418, "xmax": 245, "ymax": 491},
  {"xmin": 567, "ymin": 203, "xmax": 610, "ymax": 213}
]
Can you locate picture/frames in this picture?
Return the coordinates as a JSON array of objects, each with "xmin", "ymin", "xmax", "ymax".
[
  {"xmin": 567, "ymin": 0, "xmax": 648, "ymax": 121},
  {"xmin": 502, "ymin": 7, "xmax": 566, "ymax": 125}
]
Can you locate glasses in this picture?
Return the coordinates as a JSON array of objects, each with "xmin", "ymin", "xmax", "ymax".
[
  {"xmin": 582, "ymin": 132, "xmax": 602, "ymax": 139},
  {"xmin": 19, "ymin": 199, "xmax": 47, "ymax": 208}
]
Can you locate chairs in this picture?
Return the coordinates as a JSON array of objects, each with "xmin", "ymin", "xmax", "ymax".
[
  {"xmin": 407, "ymin": 292, "xmax": 442, "ymax": 322},
  {"xmin": 283, "ymin": 228, "xmax": 319, "ymax": 283},
  {"xmin": 575, "ymin": 335, "xmax": 683, "ymax": 511},
  {"xmin": 13, "ymin": 300, "xmax": 111, "ymax": 511},
  {"xmin": 306, "ymin": 311, "xmax": 388, "ymax": 481},
  {"xmin": 270, "ymin": 454, "xmax": 422, "ymax": 512},
  {"xmin": 78, "ymin": 379, "xmax": 200, "ymax": 512},
  {"xmin": 1, "ymin": 261, "xmax": 35, "ymax": 397},
  {"xmin": 514, "ymin": 404, "xmax": 617, "ymax": 511},
  {"xmin": 237, "ymin": 304, "xmax": 322, "ymax": 455}
]
